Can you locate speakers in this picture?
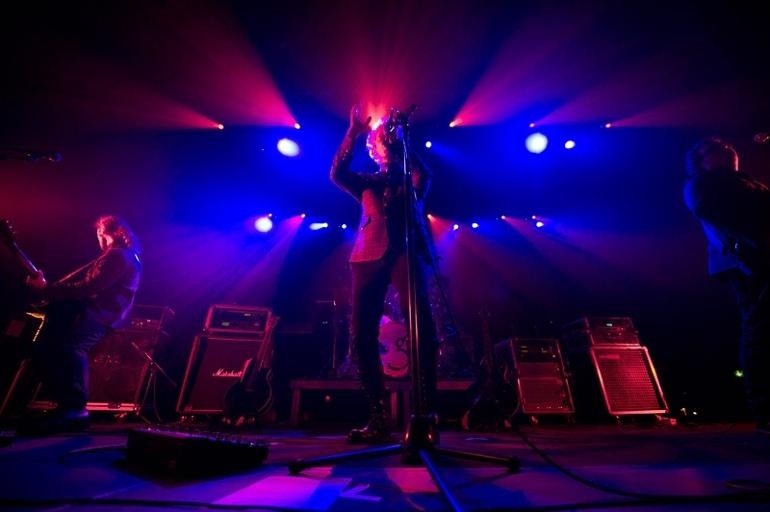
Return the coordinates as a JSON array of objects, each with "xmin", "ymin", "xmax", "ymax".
[
  {"xmin": 493, "ymin": 338, "xmax": 576, "ymax": 415},
  {"xmin": 586, "ymin": 316, "xmax": 670, "ymax": 415},
  {"xmin": 24, "ymin": 303, "xmax": 170, "ymax": 412},
  {"xmin": 176, "ymin": 304, "xmax": 272, "ymax": 414}
]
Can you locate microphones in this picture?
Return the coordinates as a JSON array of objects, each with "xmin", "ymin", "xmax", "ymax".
[
  {"xmin": 386, "ymin": 103, "xmax": 417, "ymax": 133},
  {"xmin": 33, "ymin": 150, "xmax": 64, "ymax": 163},
  {"xmin": 754, "ymin": 132, "xmax": 770, "ymax": 144}
]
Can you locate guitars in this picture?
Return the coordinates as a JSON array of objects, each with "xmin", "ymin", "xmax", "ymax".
[
  {"xmin": 477, "ymin": 309, "xmax": 519, "ymax": 419},
  {"xmin": 1, "ymin": 220, "xmax": 81, "ymax": 328},
  {"xmin": 224, "ymin": 310, "xmax": 279, "ymax": 420}
]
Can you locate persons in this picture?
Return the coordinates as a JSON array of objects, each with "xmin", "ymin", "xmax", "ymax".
[
  {"xmin": 23, "ymin": 212, "xmax": 141, "ymax": 438},
  {"xmin": 675, "ymin": 130, "xmax": 769, "ymax": 433},
  {"xmin": 325, "ymin": 103, "xmax": 440, "ymax": 466}
]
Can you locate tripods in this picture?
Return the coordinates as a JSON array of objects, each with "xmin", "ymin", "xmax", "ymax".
[{"xmin": 287, "ymin": 127, "xmax": 522, "ymax": 512}]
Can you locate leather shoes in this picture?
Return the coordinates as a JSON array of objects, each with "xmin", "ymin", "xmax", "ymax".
[{"xmin": 346, "ymin": 425, "xmax": 390, "ymax": 442}]
